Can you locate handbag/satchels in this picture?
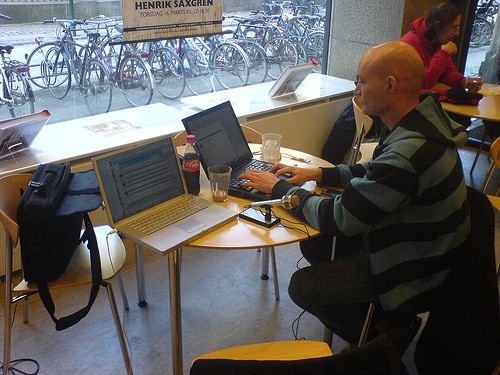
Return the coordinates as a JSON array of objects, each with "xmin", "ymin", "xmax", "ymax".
[{"xmin": 17, "ymin": 163, "xmax": 103, "ymax": 282}]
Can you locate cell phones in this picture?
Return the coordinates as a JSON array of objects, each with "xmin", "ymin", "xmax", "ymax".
[
  {"xmin": 239, "ymin": 206, "xmax": 281, "ymax": 228},
  {"xmin": 321, "ymin": 187, "xmax": 342, "ymax": 197}
]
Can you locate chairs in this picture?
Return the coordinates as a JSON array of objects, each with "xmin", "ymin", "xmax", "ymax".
[
  {"xmin": 170, "ymin": 125, "xmax": 283, "ymax": 306},
  {"xmin": 189, "ymin": 316, "xmax": 422, "ymax": 375},
  {"xmin": 0, "ymin": 173, "xmax": 136, "ymax": 374},
  {"xmin": 349, "ymin": 95, "xmax": 380, "ymax": 165},
  {"xmin": 480, "ymin": 134, "xmax": 500, "ymax": 197},
  {"xmin": 360, "ymin": 187, "xmax": 500, "ymax": 347}
]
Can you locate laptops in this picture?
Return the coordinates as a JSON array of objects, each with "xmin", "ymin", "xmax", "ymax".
[
  {"xmin": 90, "ymin": 135, "xmax": 239, "ymax": 257},
  {"xmin": 182, "ymin": 101, "xmax": 307, "ymax": 202}
]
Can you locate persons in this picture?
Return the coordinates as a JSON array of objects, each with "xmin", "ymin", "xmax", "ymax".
[
  {"xmin": 400, "ymin": 2, "xmax": 484, "ymax": 128},
  {"xmin": 237, "ymin": 41, "xmax": 474, "ymax": 357}
]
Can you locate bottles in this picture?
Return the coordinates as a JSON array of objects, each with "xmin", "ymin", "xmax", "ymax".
[{"xmin": 181, "ymin": 135, "xmax": 201, "ymax": 196}]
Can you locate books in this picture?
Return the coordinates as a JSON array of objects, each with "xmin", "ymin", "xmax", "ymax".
[
  {"xmin": 0, "ymin": 109, "xmax": 52, "ymax": 159},
  {"xmin": 267, "ymin": 64, "xmax": 315, "ymax": 97}
]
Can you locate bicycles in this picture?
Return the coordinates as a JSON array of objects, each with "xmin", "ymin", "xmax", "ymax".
[
  {"xmin": 25, "ymin": 0, "xmax": 330, "ymax": 117},
  {"xmin": 0, "ymin": 12, "xmax": 37, "ymax": 118},
  {"xmin": 466, "ymin": 0, "xmax": 500, "ymax": 47}
]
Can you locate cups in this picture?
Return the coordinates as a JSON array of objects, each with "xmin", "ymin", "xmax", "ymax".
[
  {"xmin": 207, "ymin": 164, "xmax": 232, "ymax": 204},
  {"xmin": 262, "ymin": 131, "xmax": 282, "ymax": 164},
  {"xmin": 466, "ymin": 75, "xmax": 481, "ymax": 94}
]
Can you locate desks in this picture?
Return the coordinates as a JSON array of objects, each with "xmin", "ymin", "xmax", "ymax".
[
  {"xmin": 131, "ymin": 142, "xmax": 350, "ymax": 375},
  {"xmin": 440, "ymin": 82, "xmax": 500, "ymax": 177}
]
